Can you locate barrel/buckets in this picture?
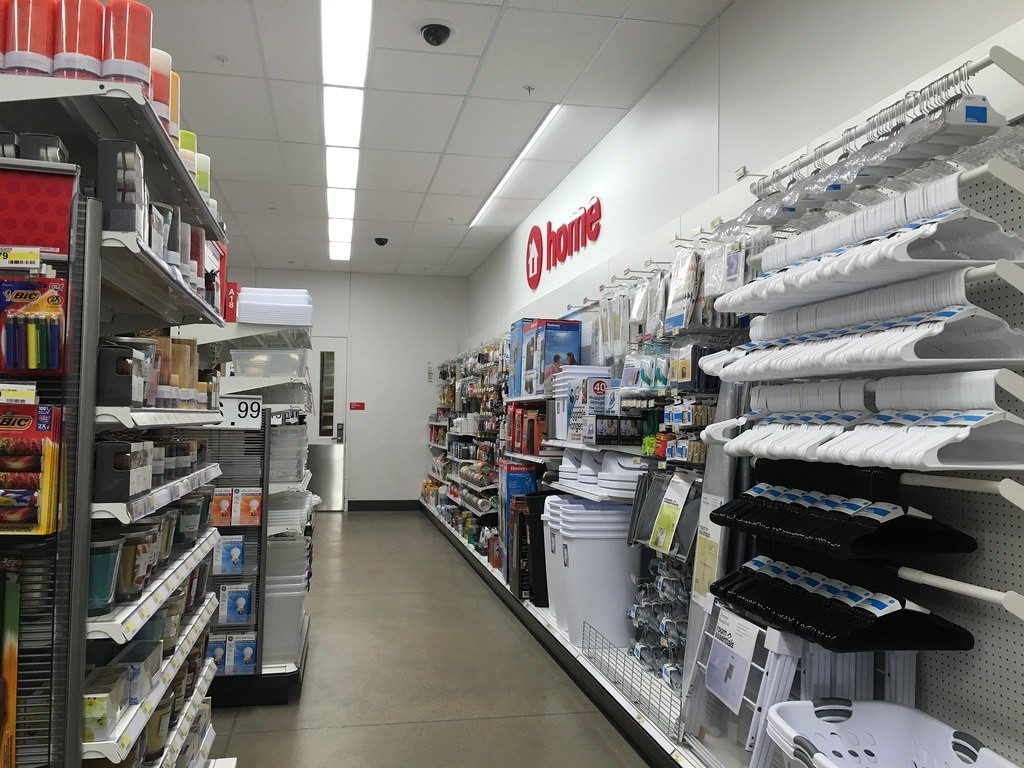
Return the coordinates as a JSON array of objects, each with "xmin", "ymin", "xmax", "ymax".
[{"xmin": 15, "ymin": 482, "xmax": 213, "ymax": 768}]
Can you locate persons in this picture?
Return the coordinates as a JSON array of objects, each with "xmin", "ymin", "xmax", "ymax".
[
  {"xmin": 622, "ymin": 420, "xmax": 640, "ymax": 436},
  {"xmin": 598, "ymin": 419, "xmax": 616, "ymax": 436},
  {"xmin": 544, "ymin": 355, "xmax": 562, "ymax": 379},
  {"xmin": 566, "ymin": 352, "xmax": 576, "ymax": 365}
]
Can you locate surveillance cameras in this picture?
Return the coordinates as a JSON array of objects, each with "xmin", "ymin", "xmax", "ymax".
[
  {"xmin": 375, "ymin": 238, "xmax": 388, "ymax": 246},
  {"xmin": 421, "ymin": 24, "xmax": 451, "ymax": 45}
]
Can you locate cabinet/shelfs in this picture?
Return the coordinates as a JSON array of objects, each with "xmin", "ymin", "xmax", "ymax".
[
  {"xmin": 426, "ymin": 395, "xmax": 666, "ymax": 518},
  {"xmin": 0, "ymin": 75, "xmax": 318, "ymax": 767}
]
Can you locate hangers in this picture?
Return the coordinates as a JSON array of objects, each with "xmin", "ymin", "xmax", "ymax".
[{"xmin": 698, "ymin": 59, "xmax": 1023, "ymax": 652}]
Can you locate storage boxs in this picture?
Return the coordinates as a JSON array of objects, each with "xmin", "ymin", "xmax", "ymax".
[
  {"xmin": 95, "ymin": 340, "xmax": 146, "ymax": 409},
  {"xmin": 497, "ymin": 318, "xmax": 583, "ymax": 600},
  {"xmin": 94, "ymin": 438, "xmax": 144, "ymax": 504},
  {"xmin": 144, "ymin": 441, "xmax": 153, "ymax": 497}
]
